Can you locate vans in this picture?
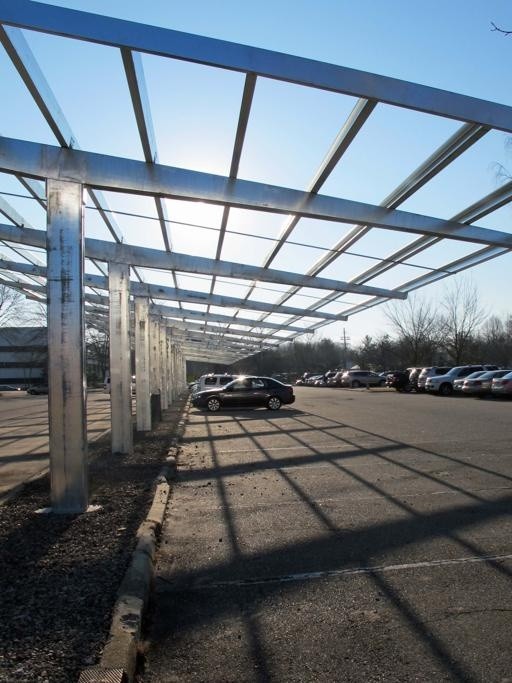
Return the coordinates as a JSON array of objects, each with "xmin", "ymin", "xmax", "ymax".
[{"xmin": 199, "ymin": 374, "xmax": 254, "ymax": 397}]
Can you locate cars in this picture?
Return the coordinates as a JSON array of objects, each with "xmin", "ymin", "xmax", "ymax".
[
  {"xmin": 0, "ymin": 376, "xmax": 136, "ymax": 395},
  {"xmin": 385, "ymin": 364, "xmax": 512, "ymax": 398},
  {"xmin": 342, "ymin": 370, "xmax": 386, "ymax": 388},
  {"xmin": 192, "ymin": 376, "xmax": 296, "ymax": 411},
  {"xmin": 297, "ymin": 370, "xmax": 340, "ymax": 388}
]
